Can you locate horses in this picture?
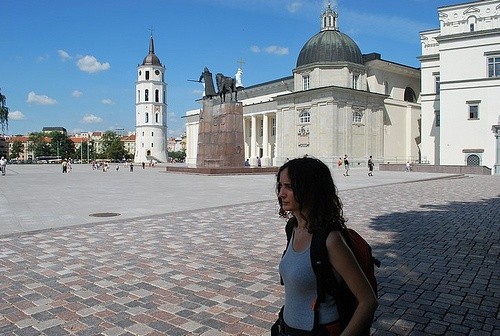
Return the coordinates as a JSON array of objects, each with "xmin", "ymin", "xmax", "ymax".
[{"xmin": 215, "ymin": 73, "xmax": 238, "ymax": 103}]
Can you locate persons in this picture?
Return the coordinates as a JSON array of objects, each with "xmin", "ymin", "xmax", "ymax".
[
  {"xmin": 274, "ymin": 158, "xmax": 378, "ymax": 336},
  {"xmin": 198, "ymin": 67, "xmax": 215, "ymax": 97},
  {"xmin": 0, "ymin": 156, "xmax": 146, "ymax": 176},
  {"xmin": 245, "ymin": 154, "xmax": 413, "ymax": 177}
]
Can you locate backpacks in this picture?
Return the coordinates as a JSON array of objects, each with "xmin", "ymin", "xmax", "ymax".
[{"xmin": 285, "ymin": 216, "xmax": 381, "ymax": 328}]
